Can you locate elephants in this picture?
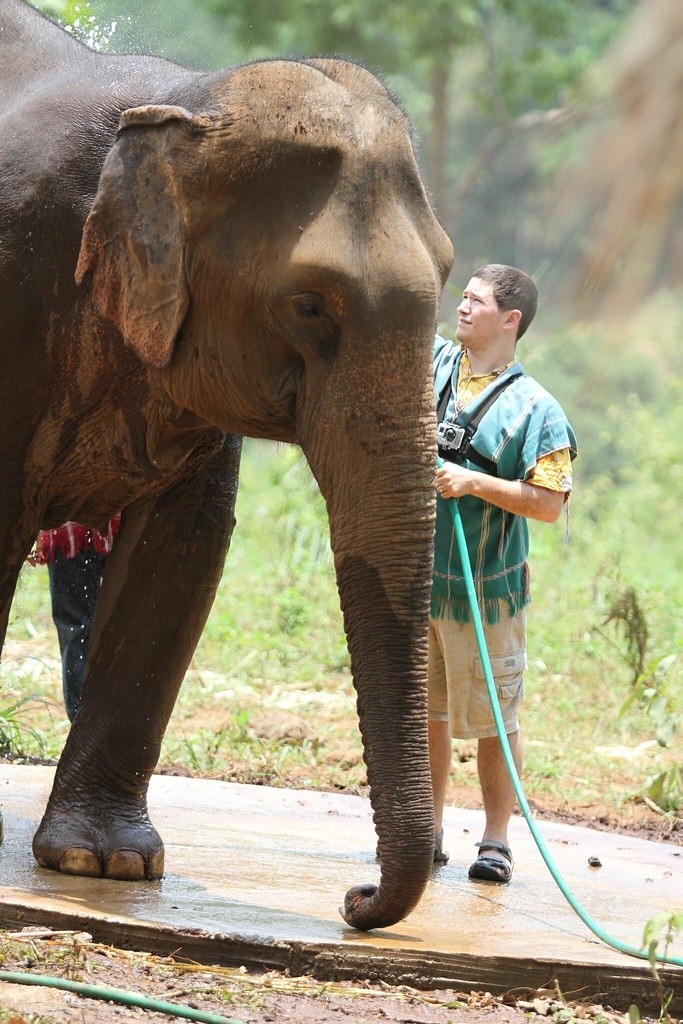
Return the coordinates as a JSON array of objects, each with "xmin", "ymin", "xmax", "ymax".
[{"xmin": 0, "ymin": 0, "xmax": 457, "ymax": 933}]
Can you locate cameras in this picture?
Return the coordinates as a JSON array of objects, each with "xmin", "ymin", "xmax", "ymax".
[{"xmin": 437, "ymin": 421, "xmax": 465, "ymax": 450}]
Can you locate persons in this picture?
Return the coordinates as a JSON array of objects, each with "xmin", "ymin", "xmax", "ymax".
[
  {"xmin": 427, "ymin": 264, "xmax": 577, "ymax": 879},
  {"xmin": 27, "ymin": 509, "xmax": 122, "ymax": 726}
]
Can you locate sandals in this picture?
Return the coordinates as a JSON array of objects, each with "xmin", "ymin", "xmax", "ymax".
[
  {"xmin": 433, "ymin": 827, "xmax": 450, "ymax": 863},
  {"xmin": 468, "ymin": 840, "xmax": 514, "ymax": 881}
]
